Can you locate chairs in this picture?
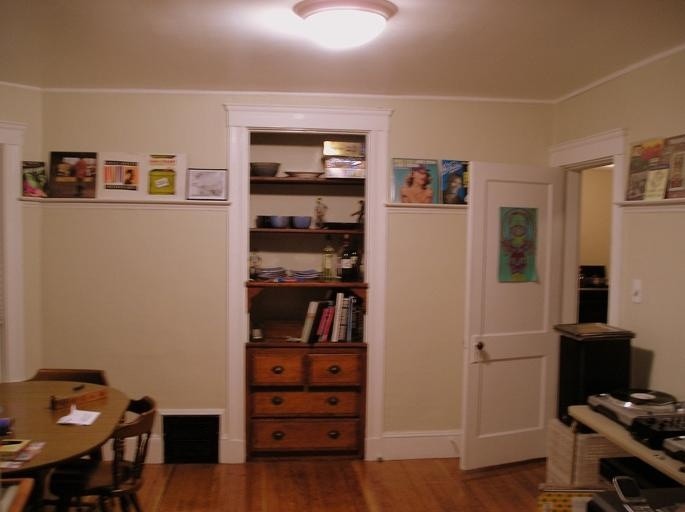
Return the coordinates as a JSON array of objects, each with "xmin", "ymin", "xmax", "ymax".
[{"xmin": 0, "ymin": 368, "xmax": 158, "ymax": 512}]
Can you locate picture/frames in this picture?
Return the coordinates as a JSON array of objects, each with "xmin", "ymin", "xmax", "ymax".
[{"xmin": 184, "ymin": 167, "xmax": 228, "ymax": 201}]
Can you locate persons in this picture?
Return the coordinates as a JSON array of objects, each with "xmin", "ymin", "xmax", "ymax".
[
  {"xmin": 125, "ymin": 169, "xmax": 134, "ymax": 184},
  {"xmin": 400, "ymin": 166, "xmax": 433, "ymax": 204},
  {"xmin": 443, "ymin": 172, "xmax": 468, "ymax": 204},
  {"xmin": 73, "ymin": 157, "xmax": 87, "ymax": 194}
]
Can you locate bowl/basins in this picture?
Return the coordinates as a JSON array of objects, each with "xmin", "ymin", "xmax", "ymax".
[
  {"xmin": 249, "ymin": 162, "xmax": 281, "ymax": 178},
  {"xmin": 256, "ymin": 215, "xmax": 313, "ymax": 229}
]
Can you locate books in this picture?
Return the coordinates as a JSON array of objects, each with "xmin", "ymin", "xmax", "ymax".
[
  {"xmin": 551, "ymin": 320, "xmax": 636, "ymax": 342},
  {"xmin": 0, "ymin": 438, "xmax": 32, "ymax": 461},
  {"xmin": 300, "ymin": 291, "xmax": 364, "ymax": 345}
]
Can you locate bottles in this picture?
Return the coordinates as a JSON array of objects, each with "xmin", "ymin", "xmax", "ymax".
[{"xmin": 323, "ymin": 234, "xmax": 360, "ymax": 281}]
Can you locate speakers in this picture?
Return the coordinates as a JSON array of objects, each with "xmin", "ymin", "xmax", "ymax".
[{"xmin": 558, "ymin": 334, "xmax": 631, "ymax": 434}]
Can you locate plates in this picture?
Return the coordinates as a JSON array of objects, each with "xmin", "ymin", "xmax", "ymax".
[
  {"xmin": 283, "ymin": 170, "xmax": 324, "ymax": 179},
  {"xmin": 317, "ymin": 221, "xmax": 364, "ymax": 230}
]
